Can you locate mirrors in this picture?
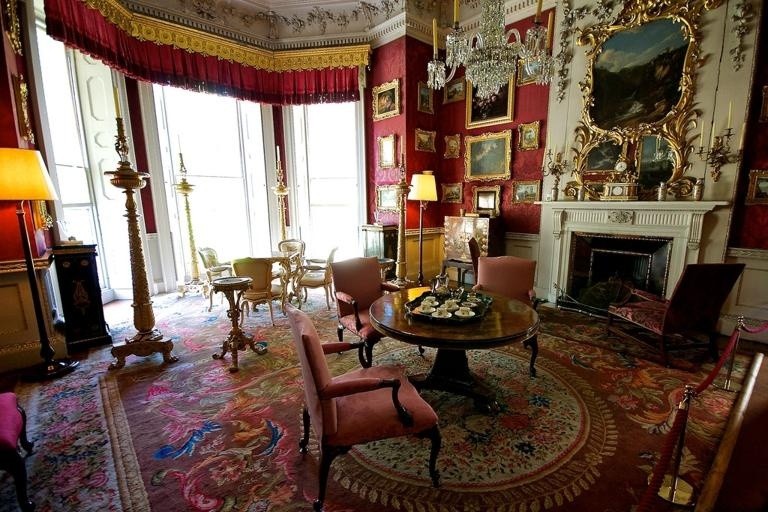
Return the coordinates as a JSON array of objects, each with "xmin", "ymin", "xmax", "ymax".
[
  {"xmin": 576, "ymin": 1, "xmax": 707, "ymax": 134},
  {"xmin": 568, "ymin": 130, "xmax": 686, "ymax": 196}
]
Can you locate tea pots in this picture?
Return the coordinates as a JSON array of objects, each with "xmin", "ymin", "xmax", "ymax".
[{"xmin": 432, "ymin": 273, "xmax": 450, "ymax": 302}]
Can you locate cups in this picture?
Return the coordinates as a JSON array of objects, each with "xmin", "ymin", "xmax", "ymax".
[
  {"xmin": 462, "ymin": 299, "xmax": 471, "ymax": 307},
  {"xmin": 437, "ymin": 308, "xmax": 448, "ymax": 319},
  {"xmin": 467, "ymin": 293, "xmax": 477, "ymax": 301},
  {"xmin": 425, "ymin": 297, "xmax": 435, "ymax": 306},
  {"xmin": 459, "ymin": 307, "xmax": 470, "ymax": 317},
  {"xmin": 445, "ymin": 300, "xmax": 455, "ymax": 311},
  {"xmin": 420, "ymin": 301, "xmax": 433, "ymax": 313}
]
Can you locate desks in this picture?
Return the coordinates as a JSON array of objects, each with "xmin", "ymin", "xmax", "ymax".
[
  {"xmin": 209, "ymin": 270, "xmax": 267, "ymax": 373},
  {"xmin": 244, "ymin": 252, "xmax": 304, "ymax": 314}
]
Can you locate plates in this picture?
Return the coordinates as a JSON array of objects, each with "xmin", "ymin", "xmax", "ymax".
[
  {"xmin": 431, "ymin": 311, "xmax": 452, "ymax": 318},
  {"xmin": 418, "ymin": 306, "xmax": 436, "ymax": 313},
  {"xmin": 462, "ymin": 303, "xmax": 478, "ymax": 309},
  {"xmin": 455, "ymin": 311, "xmax": 476, "ymax": 318},
  {"xmin": 420, "ymin": 300, "xmax": 439, "ymax": 306},
  {"xmin": 467, "ymin": 297, "xmax": 481, "ymax": 303},
  {"xmin": 440, "ymin": 304, "xmax": 460, "ymax": 311}
]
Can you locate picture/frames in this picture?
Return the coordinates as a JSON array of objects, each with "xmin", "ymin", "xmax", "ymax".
[
  {"xmin": 463, "ymin": 70, "xmax": 516, "ymax": 130},
  {"xmin": 746, "ymin": 169, "xmax": 768, "ymax": 207},
  {"xmin": 416, "ymin": 81, "xmax": 436, "ymax": 115},
  {"xmin": 443, "ymin": 132, "xmax": 460, "ymax": 160},
  {"xmin": 514, "ymin": 59, "xmax": 550, "ymax": 87},
  {"xmin": 462, "ymin": 131, "xmax": 512, "ymax": 182},
  {"xmin": 375, "ymin": 133, "xmax": 399, "ymax": 170},
  {"xmin": 440, "ymin": 74, "xmax": 466, "ymax": 105},
  {"xmin": 518, "ymin": 120, "xmax": 539, "ymax": 153},
  {"xmin": 372, "ymin": 78, "xmax": 401, "ymax": 123},
  {"xmin": 438, "ymin": 182, "xmax": 464, "ymax": 206},
  {"xmin": 378, "ymin": 185, "xmax": 399, "ymax": 216},
  {"xmin": 414, "ymin": 129, "xmax": 437, "ymax": 153},
  {"xmin": 509, "ymin": 179, "xmax": 540, "ymax": 207},
  {"xmin": 471, "ymin": 184, "xmax": 501, "ymax": 215}
]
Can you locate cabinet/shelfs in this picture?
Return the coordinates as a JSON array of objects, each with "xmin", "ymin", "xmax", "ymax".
[
  {"xmin": 358, "ymin": 226, "xmax": 400, "ymax": 283},
  {"xmin": 443, "ymin": 216, "xmax": 502, "ymax": 272}
]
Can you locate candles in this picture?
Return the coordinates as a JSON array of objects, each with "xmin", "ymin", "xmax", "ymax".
[
  {"xmin": 275, "ymin": 146, "xmax": 280, "ymax": 164},
  {"xmin": 728, "ymin": 101, "xmax": 732, "ymax": 128},
  {"xmin": 699, "ymin": 121, "xmax": 705, "ymax": 146},
  {"xmin": 112, "ymin": 86, "xmax": 123, "ymax": 119},
  {"xmin": 738, "ymin": 122, "xmax": 747, "ymax": 150},
  {"xmin": 711, "ymin": 121, "xmax": 715, "ymax": 143}
]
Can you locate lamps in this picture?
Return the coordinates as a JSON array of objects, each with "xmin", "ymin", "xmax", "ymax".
[
  {"xmin": 0, "ymin": 147, "xmax": 82, "ymax": 382},
  {"xmin": 407, "ymin": 174, "xmax": 437, "ymax": 285},
  {"xmin": 427, "ymin": 1, "xmax": 551, "ymax": 97}
]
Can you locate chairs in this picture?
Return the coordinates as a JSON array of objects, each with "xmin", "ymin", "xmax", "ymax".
[
  {"xmin": 326, "ymin": 254, "xmax": 428, "ymax": 364},
  {"xmin": 199, "ymin": 247, "xmax": 234, "ymax": 309},
  {"xmin": 293, "ymin": 247, "xmax": 338, "ymax": 311},
  {"xmin": 278, "ymin": 240, "xmax": 305, "ymax": 258},
  {"xmin": 231, "ymin": 257, "xmax": 277, "ymax": 328},
  {"xmin": 0, "ymin": 391, "xmax": 40, "ymax": 511},
  {"xmin": 472, "ymin": 252, "xmax": 540, "ymax": 377},
  {"xmin": 609, "ymin": 260, "xmax": 747, "ymax": 369},
  {"xmin": 280, "ymin": 301, "xmax": 446, "ymax": 506}
]
